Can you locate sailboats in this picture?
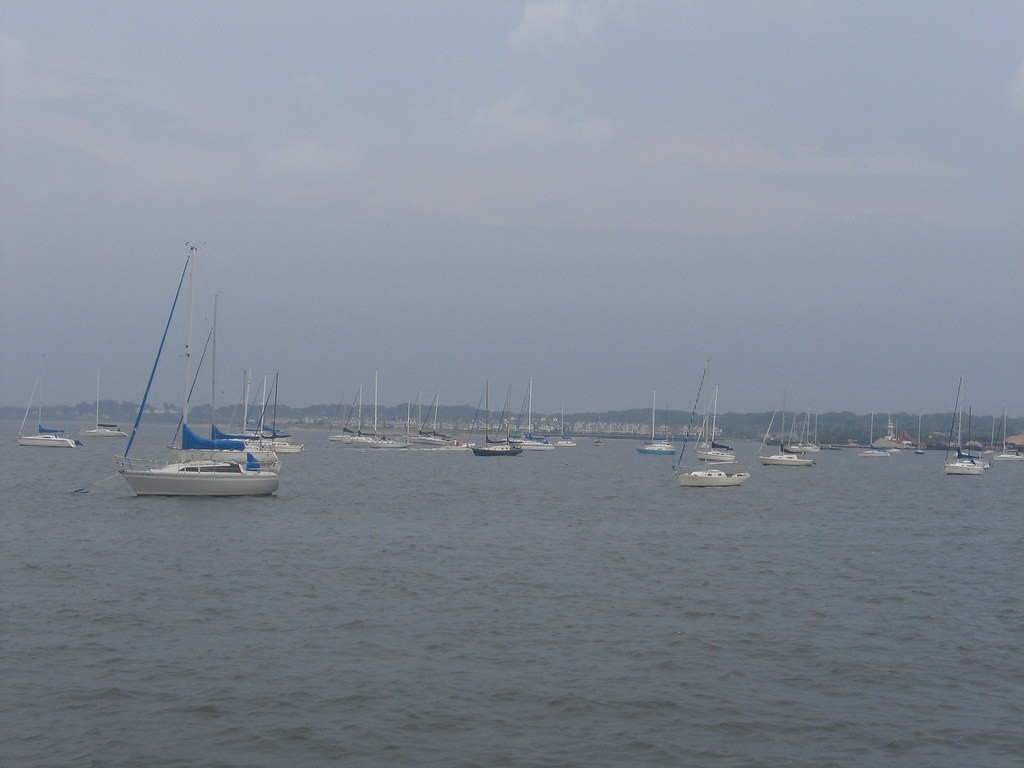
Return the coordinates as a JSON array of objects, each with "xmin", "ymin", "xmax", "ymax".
[
  {"xmin": 694, "ymin": 385, "xmax": 735, "ymax": 461},
  {"xmin": 635, "ymin": 391, "xmax": 676, "ymax": 453},
  {"xmin": 554, "ymin": 400, "xmax": 576, "ymax": 446},
  {"xmin": 993, "ymin": 404, "xmax": 1024, "ymax": 461},
  {"xmin": 82, "ymin": 367, "xmax": 128, "ymax": 436},
  {"xmin": 17, "ymin": 353, "xmax": 85, "ymax": 449},
  {"xmin": 164, "ymin": 293, "xmax": 304, "ymax": 470},
  {"xmin": 944, "ymin": 376, "xmax": 995, "ymax": 474},
  {"xmin": 855, "ymin": 412, "xmax": 890, "ymax": 457},
  {"xmin": 329, "ymin": 370, "xmax": 414, "ymax": 448},
  {"xmin": 914, "ymin": 414, "xmax": 924, "ymax": 454},
  {"xmin": 673, "ymin": 358, "xmax": 750, "ymax": 488},
  {"xmin": 404, "ymin": 391, "xmax": 455, "ymax": 446},
  {"xmin": 467, "ymin": 377, "xmax": 555, "ymax": 455},
  {"xmin": 784, "ymin": 399, "xmax": 820, "ymax": 451},
  {"xmin": 119, "ymin": 242, "xmax": 279, "ymax": 497},
  {"xmin": 886, "ymin": 420, "xmax": 900, "ymax": 452},
  {"xmin": 755, "ymin": 388, "xmax": 814, "ymax": 466},
  {"xmin": 592, "ymin": 416, "xmax": 603, "ymax": 445}
]
